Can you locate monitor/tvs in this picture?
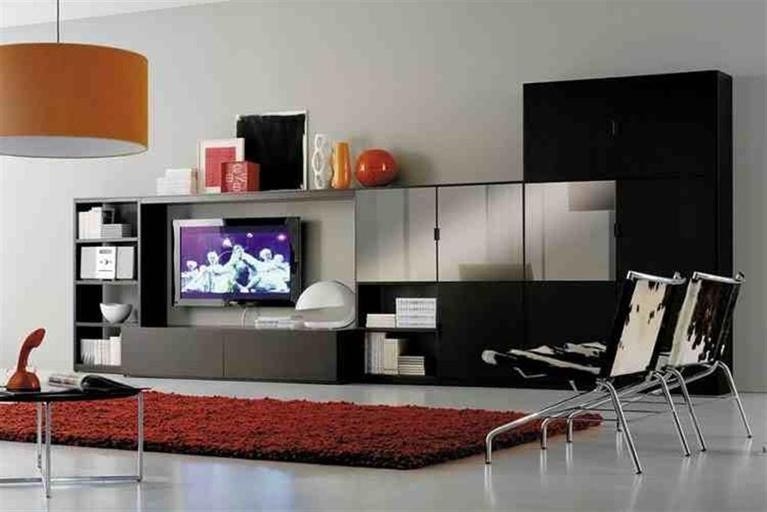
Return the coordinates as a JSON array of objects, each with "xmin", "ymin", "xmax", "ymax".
[{"xmin": 168, "ymin": 216, "xmax": 303, "ymax": 310}]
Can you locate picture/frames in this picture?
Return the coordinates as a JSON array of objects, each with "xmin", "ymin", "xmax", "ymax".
[
  {"xmin": 199, "ymin": 138, "xmax": 245, "ymax": 192},
  {"xmin": 231, "ymin": 109, "xmax": 308, "ymax": 190}
]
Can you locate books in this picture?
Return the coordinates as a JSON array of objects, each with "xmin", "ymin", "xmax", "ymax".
[
  {"xmin": 156, "ymin": 169, "xmax": 197, "ymax": 196},
  {"xmin": 365, "ymin": 333, "xmax": 426, "ymax": 376},
  {"xmin": 78, "ymin": 207, "xmax": 131, "ymax": 240},
  {"xmin": 365, "ymin": 295, "xmax": 436, "ymax": 329},
  {"xmin": 80, "ymin": 336, "xmax": 122, "ymax": 365}
]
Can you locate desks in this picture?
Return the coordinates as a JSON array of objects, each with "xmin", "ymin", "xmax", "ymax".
[{"xmin": 1, "ymin": 384, "xmax": 151, "ymax": 499}]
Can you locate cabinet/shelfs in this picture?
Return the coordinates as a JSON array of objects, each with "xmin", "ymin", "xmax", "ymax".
[
  {"xmin": 522, "ymin": 71, "xmax": 734, "ymax": 180},
  {"xmin": 124, "ymin": 327, "xmax": 343, "ymax": 385},
  {"xmin": 72, "ymin": 199, "xmax": 138, "ymax": 374}
]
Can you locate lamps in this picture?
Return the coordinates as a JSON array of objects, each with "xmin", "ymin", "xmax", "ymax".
[
  {"xmin": 6, "ymin": 328, "xmax": 46, "ymax": 390},
  {"xmin": 1, "ymin": 1, "xmax": 149, "ymax": 160}
]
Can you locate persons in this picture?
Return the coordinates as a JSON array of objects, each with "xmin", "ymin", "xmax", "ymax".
[{"xmin": 179, "ymin": 237, "xmax": 291, "ymax": 295}]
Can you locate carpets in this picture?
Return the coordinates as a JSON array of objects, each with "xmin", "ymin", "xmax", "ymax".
[{"xmin": 2, "ymin": 385, "xmax": 605, "ymax": 471}]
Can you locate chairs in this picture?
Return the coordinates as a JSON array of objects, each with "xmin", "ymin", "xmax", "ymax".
[
  {"xmin": 567, "ymin": 269, "xmax": 753, "ymax": 459},
  {"xmin": 481, "ymin": 267, "xmax": 685, "ymax": 478}
]
